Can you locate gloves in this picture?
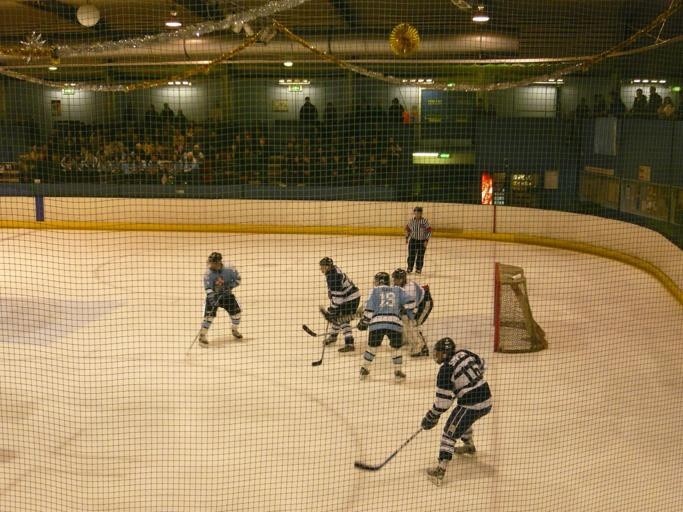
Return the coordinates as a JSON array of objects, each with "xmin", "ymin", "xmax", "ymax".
[
  {"xmin": 328, "ymin": 306, "xmax": 339, "ymax": 322},
  {"xmin": 208, "ymin": 297, "xmax": 219, "ymax": 307},
  {"xmin": 356, "ymin": 318, "xmax": 370, "ymax": 331},
  {"xmin": 421, "ymin": 410, "xmax": 440, "ymax": 430}
]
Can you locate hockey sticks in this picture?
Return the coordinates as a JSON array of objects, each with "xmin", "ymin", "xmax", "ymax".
[
  {"xmin": 311, "ymin": 301, "xmax": 332, "ymax": 367},
  {"xmin": 301, "ymin": 324, "xmax": 359, "ymax": 337},
  {"xmin": 353, "ymin": 393, "xmax": 457, "ymax": 472},
  {"xmin": 183, "ymin": 297, "xmax": 223, "ymax": 359}
]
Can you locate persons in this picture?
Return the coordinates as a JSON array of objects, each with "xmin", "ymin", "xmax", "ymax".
[
  {"xmin": 319, "ymin": 256, "xmax": 360, "ymax": 352},
  {"xmin": 656, "ymin": 96, "xmax": 675, "ymax": 119},
  {"xmin": 404, "ymin": 206, "xmax": 433, "ymax": 274},
  {"xmin": 392, "ymin": 268, "xmax": 434, "ymax": 356},
  {"xmin": 607, "ymin": 90, "xmax": 626, "ymax": 111},
  {"xmin": 643, "ymin": 85, "xmax": 663, "ymax": 113},
  {"xmin": 357, "ymin": 272, "xmax": 416, "ymax": 379},
  {"xmin": 421, "ymin": 338, "xmax": 493, "ymax": 480},
  {"xmin": 629, "ymin": 88, "xmax": 648, "ymax": 112},
  {"xmin": 199, "ymin": 252, "xmax": 243, "ymax": 344},
  {"xmin": 576, "ymin": 96, "xmax": 591, "ymax": 116},
  {"xmin": 593, "ymin": 93, "xmax": 606, "ymax": 113},
  {"xmin": 675, "ymin": 90, "xmax": 683, "ymax": 120},
  {"xmin": 0, "ymin": 98, "xmax": 498, "ymax": 207}
]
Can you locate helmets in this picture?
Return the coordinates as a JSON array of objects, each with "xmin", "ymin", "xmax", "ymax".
[
  {"xmin": 207, "ymin": 251, "xmax": 222, "ymax": 263},
  {"xmin": 374, "ymin": 272, "xmax": 390, "ymax": 286},
  {"xmin": 319, "ymin": 257, "xmax": 333, "ymax": 266},
  {"xmin": 391, "ymin": 268, "xmax": 406, "ymax": 279},
  {"xmin": 432, "ymin": 337, "xmax": 455, "ymax": 364}
]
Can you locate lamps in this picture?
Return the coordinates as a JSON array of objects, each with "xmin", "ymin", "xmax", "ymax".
[
  {"xmin": 473, "ymin": 5, "xmax": 490, "ymax": 23},
  {"xmin": 77, "ymin": 0, "xmax": 100, "ymax": 27}
]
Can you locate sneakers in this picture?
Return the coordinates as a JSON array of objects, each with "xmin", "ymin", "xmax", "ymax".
[
  {"xmin": 337, "ymin": 345, "xmax": 353, "ymax": 352},
  {"xmin": 198, "ymin": 334, "xmax": 208, "ymax": 344},
  {"xmin": 415, "ymin": 268, "xmax": 421, "ymax": 273},
  {"xmin": 426, "ymin": 466, "xmax": 446, "ymax": 479},
  {"xmin": 410, "ymin": 347, "xmax": 428, "ymax": 357},
  {"xmin": 231, "ymin": 330, "xmax": 242, "ymax": 338},
  {"xmin": 322, "ymin": 336, "xmax": 337, "ymax": 343},
  {"xmin": 359, "ymin": 366, "xmax": 370, "ymax": 375},
  {"xmin": 406, "ymin": 267, "xmax": 413, "ymax": 273},
  {"xmin": 454, "ymin": 446, "xmax": 475, "ymax": 454},
  {"xmin": 393, "ymin": 370, "xmax": 406, "ymax": 378}
]
